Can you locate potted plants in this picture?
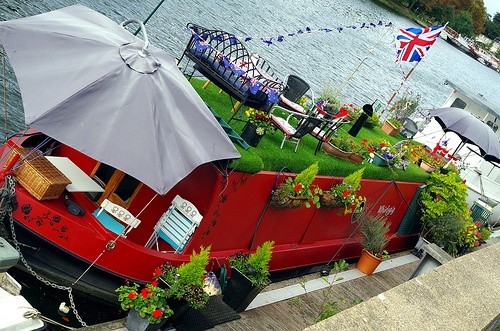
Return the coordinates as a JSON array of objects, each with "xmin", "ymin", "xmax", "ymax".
[
  {"xmin": 381, "ymin": 117, "xmax": 404, "ymax": 138},
  {"xmin": 413, "ymin": 165, "xmax": 475, "ymax": 252},
  {"xmin": 347, "ymin": 198, "xmax": 400, "ymax": 277},
  {"xmin": 363, "ymin": 110, "xmax": 381, "ymax": 131},
  {"xmin": 320, "ymin": 135, "xmax": 367, "ymax": 165}
]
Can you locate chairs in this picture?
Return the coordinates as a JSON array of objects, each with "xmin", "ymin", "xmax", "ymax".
[
  {"xmin": 269, "ymin": 110, "xmax": 325, "ymax": 154},
  {"xmin": 309, "ymin": 114, "xmax": 353, "ymax": 160},
  {"xmin": 142, "ymin": 194, "xmax": 204, "ymax": 255},
  {"xmin": 93, "ymin": 198, "xmax": 141, "ymax": 239},
  {"xmin": 278, "ymin": 74, "xmax": 315, "ymax": 118}
]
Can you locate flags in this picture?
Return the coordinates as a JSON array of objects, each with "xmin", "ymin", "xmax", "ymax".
[{"xmin": 394, "ymin": 25, "xmax": 447, "ymax": 63}]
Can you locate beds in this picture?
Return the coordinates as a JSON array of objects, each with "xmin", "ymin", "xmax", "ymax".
[{"xmin": 176, "ymin": 20, "xmax": 291, "ymax": 130}]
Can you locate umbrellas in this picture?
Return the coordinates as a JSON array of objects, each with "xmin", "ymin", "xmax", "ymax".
[
  {"xmin": 0, "ymin": 2, "xmax": 243, "ymax": 196},
  {"xmin": 428, "ymin": 106, "xmax": 500, "ymax": 170}
]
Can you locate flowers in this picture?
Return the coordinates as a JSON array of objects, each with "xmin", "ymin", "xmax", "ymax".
[
  {"xmin": 115, "ymin": 266, "xmax": 174, "ymax": 326},
  {"xmin": 279, "ymin": 161, "xmax": 323, "ymax": 211},
  {"xmin": 225, "ymin": 240, "xmax": 276, "ymax": 290},
  {"xmin": 155, "ymin": 243, "xmax": 212, "ymax": 310},
  {"xmin": 362, "ymin": 137, "xmax": 459, "ymax": 165},
  {"xmin": 330, "ymin": 166, "xmax": 368, "ymax": 214},
  {"xmin": 244, "ymin": 108, "xmax": 277, "ymax": 135}
]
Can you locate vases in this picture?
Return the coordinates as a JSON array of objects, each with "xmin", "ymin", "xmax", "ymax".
[
  {"xmin": 123, "ymin": 308, "xmax": 164, "ymax": 331},
  {"xmin": 239, "ymin": 120, "xmax": 267, "ymax": 148},
  {"xmin": 374, "ymin": 152, "xmax": 395, "ymax": 168},
  {"xmin": 420, "ymin": 160, "xmax": 438, "ymax": 173},
  {"xmin": 268, "ymin": 188, "xmax": 309, "ymax": 209},
  {"xmin": 221, "ymin": 266, "xmax": 266, "ymax": 314},
  {"xmin": 319, "ymin": 190, "xmax": 348, "ymax": 209},
  {"xmin": 157, "ymin": 277, "xmax": 190, "ymax": 321}
]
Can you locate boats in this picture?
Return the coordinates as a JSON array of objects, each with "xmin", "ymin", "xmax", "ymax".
[
  {"xmin": 401, "ymin": 77, "xmax": 500, "ymax": 227},
  {"xmin": 0, "ymin": 71, "xmax": 462, "ymax": 331},
  {"xmin": 438, "ymin": 26, "xmax": 500, "ymax": 74}
]
